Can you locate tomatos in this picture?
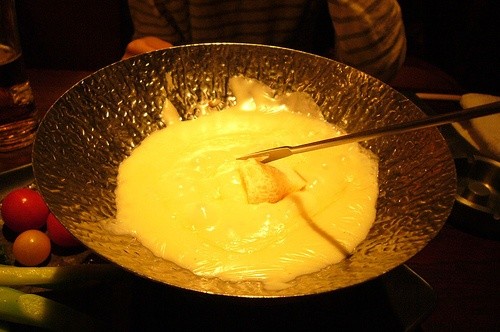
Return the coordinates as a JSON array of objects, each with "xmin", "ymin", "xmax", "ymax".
[
  {"xmin": 1, "ymin": 188, "xmax": 50, "ymax": 233},
  {"xmin": 48, "ymin": 212, "xmax": 82, "ymax": 249},
  {"xmin": 12, "ymin": 229, "xmax": 50, "ymax": 265}
]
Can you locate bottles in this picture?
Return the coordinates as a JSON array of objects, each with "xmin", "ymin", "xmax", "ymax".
[{"xmin": 0, "ymin": 30, "xmax": 39, "ymax": 153}]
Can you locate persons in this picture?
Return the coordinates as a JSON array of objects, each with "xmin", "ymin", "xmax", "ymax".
[{"xmin": 121, "ymin": 0, "xmax": 408, "ymax": 84}]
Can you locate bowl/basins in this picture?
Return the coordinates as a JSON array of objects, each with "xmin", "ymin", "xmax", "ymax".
[{"xmin": 31, "ymin": 42, "xmax": 457, "ymax": 298}]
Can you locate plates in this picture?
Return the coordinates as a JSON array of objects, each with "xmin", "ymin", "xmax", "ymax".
[{"xmin": 0, "ymin": 163, "xmax": 440, "ymax": 332}]
